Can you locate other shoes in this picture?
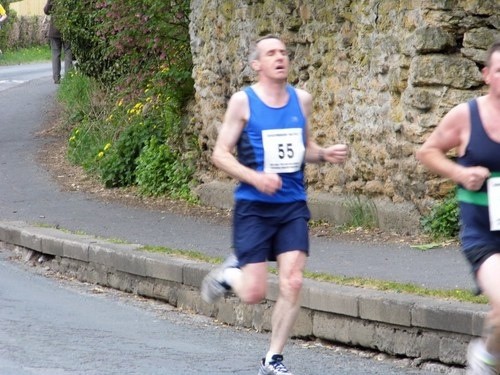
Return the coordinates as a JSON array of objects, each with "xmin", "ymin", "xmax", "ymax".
[{"xmin": 54, "ymin": 79, "xmax": 60, "ymax": 84}]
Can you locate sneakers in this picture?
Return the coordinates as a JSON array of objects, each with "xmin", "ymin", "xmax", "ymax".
[
  {"xmin": 202, "ymin": 255, "xmax": 240, "ymax": 303},
  {"xmin": 257, "ymin": 354, "xmax": 296, "ymax": 375},
  {"xmin": 466, "ymin": 337, "xmax": 500, "ymax": 374}
]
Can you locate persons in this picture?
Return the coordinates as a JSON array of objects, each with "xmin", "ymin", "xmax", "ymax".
[
  {"xmin": 44, "ymin": 0, "xmax": 73, "ymax": 83},
  {"xmin": 0, "ymin": 3, "xmax": 8, "ymax": 55},
  {"xmin": 415, "ymin": 41, "xmax": 500, "ymax": 373},
  {"xmin": 201, "ymin": 33, "xmax": 351, "ymax": 375}
]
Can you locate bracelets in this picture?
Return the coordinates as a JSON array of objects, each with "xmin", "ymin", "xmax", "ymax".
[{"xmin": 318, "ymin": 149, "xmax": 326, "ymax": 162}]
